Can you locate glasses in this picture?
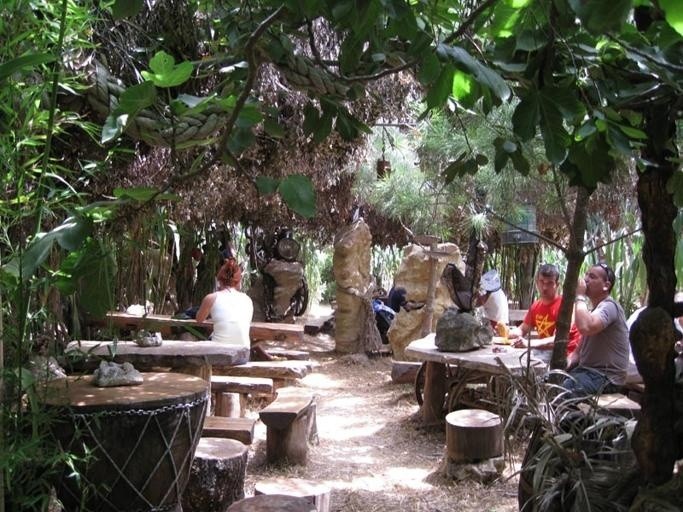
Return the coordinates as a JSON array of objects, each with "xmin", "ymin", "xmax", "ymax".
[{"xmin": 600, "ymin": 263, "xmax": 610, "ymax": 283}]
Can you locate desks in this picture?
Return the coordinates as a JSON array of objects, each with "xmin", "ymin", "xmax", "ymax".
[
  {"xmin": 28, "ymin": 313, "xmax": 305, "ymax": 512},
  {"xmin": 405, "ymin": 338, "xmax": 548, "ymax": 427}
]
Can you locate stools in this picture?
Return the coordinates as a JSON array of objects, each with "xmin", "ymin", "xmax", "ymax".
[
  {"xmin": 226, "ymin": 476, "xmax": 331, "ymax": 512},
  {"xmin": 445, "ymin": 409, "xmax": 508, "ymax": 483}
]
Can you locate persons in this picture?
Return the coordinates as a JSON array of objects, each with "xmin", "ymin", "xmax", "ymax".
[
  {"xmin": 372, "ymin": 285, "xmax": 424, "ymax": 338},
  {"xmin": 473, "ymin": 268, "xmax": 510, "ymax": 340},
  {"xmin": 623, "ymin": 292, "xmax": 683, "ymax": 384},
  {"xmin": 551, "ymin": 263, "xmax": 628, "ymax": 416},
  {"xmin": 508, "ymin": 264, "xmax": 564, "ymax": 349},
  {"xmin": 194, "ymin": 256, "xmax": 256, "ymax": 367}
]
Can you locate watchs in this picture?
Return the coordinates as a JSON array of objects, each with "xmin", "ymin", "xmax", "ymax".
[{"xmin": 573, "ymin": 296, "xmax": 584, "ymax": 302}]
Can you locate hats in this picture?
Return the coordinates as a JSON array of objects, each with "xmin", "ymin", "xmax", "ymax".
[{"xmin": 479, "ymin": 269, "xmax": 501, "ymax": 292}]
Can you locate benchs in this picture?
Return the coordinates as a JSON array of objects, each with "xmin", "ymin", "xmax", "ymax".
[
  {"xmin": 589, "ymin": 392, "xmax": 641, "ymax": 416},
  {"xmin": 213, "ymin": 359, "xmax": 319, "ymax": 466}
]
